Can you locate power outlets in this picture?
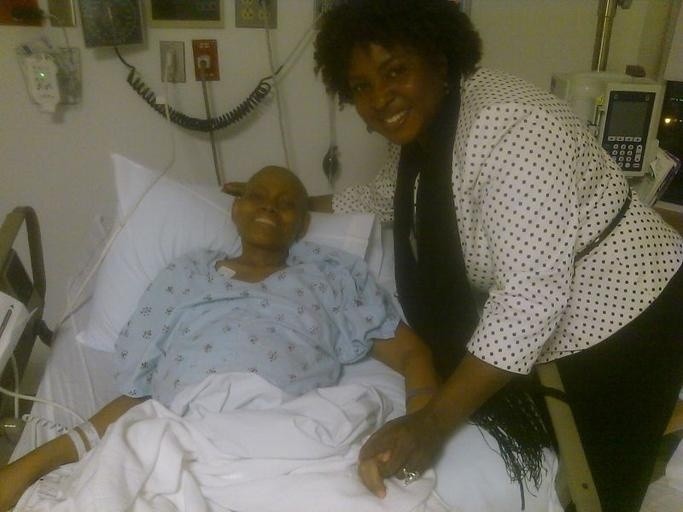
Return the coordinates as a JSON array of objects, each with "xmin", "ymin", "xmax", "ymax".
[
  {"xmin": 193, "ymin": 40, "xmax": 219, "ymax": 81},
  {"xmin": 160, "ymin": 41, "xmax": 185, "ymax": 82},
  {"xmin": 236, "ymin": 0, "xmax": 277, "ymax": 29}
]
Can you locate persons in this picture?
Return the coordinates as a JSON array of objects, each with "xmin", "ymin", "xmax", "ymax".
[
  {"xmin": 222, "ymin": 2, "xmax": 681, "ymax": 511},
  {"xmin": 1, "ymin": 163, "xmax": 440, "ymax": 510}
]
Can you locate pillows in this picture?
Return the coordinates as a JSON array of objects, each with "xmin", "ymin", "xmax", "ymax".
[{"xmin": 74, "ymin": 152, "xmax": 384, "ymax": 356}]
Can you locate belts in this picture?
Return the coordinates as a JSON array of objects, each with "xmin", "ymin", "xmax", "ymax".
[{"xmin": 575, "ymin": 188, "xmax": 631, "ymax": 262}]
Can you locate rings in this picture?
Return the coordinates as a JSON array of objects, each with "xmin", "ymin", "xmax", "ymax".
[{"xmin": 400, "ymin": 462, "xmax": 424, "ymax": 486}]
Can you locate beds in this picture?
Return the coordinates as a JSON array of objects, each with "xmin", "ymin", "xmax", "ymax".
[{"xmin": 0, "ymin": 151, "xmax": 607, "ymax": 512}]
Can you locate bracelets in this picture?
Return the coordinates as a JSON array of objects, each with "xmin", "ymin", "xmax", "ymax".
[
  {"xmin": 77, "ymin": 419, "xmax": 100, "ymax": 448},
  {"xmin": 401, "ymin": 382, "xmax": 441, "ymax": 406},
  {"xmin": 66, "ymin": 428, "xmax": 91, "ymax": 461}
]
